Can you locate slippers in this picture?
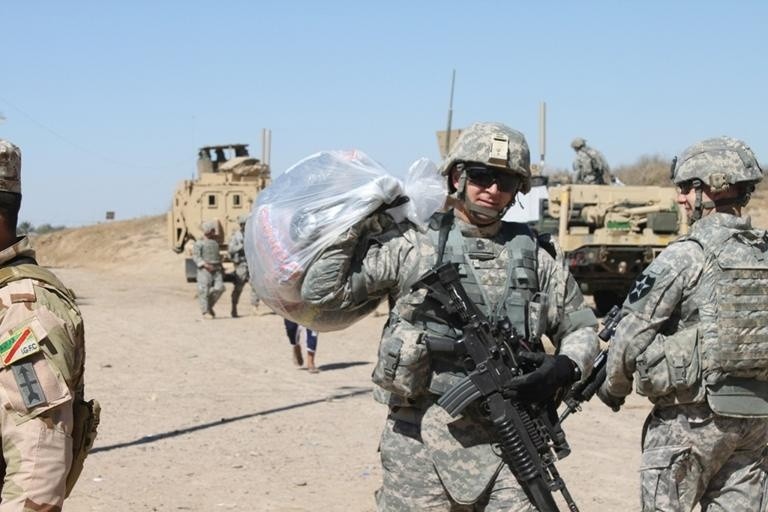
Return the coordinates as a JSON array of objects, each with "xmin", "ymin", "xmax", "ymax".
[{"xmin": 293, "ymin": 344, "xmax": 320, "ymax": 374}]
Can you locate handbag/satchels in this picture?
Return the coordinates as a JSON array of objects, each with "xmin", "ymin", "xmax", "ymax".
[
  {"xmin": 630, "ymin": 324, "xmax": 703, "ymax": 401},
  {"xmin": 370, "ymin": 325, "xmax": 438, "ymax": 401}
]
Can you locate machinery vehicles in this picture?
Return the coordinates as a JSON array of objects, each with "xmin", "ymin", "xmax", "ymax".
[
  {"xmin": 167, "ymin": 126, "xmax": 274, "ymax": 283},
  {"xmin": 435, "ymin": 68, "xmax": 693, "ymax": 320}
]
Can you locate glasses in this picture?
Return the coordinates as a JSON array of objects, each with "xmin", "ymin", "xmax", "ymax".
[{"xmin": 465, "ymin": 166, "xmax": 518, "ymax": 192}]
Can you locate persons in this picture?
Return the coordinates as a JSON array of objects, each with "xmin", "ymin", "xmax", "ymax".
[
  {"xmin": 597, "ymin": 137, "xmax": 767, "ymax": 512},
  {"xmin": 229, "ymin": 217, "xmax": 263, "ymax": 318},
  {"xmin": 301, "ymin": 122, "xmax": 601, "ymax": 512},
  {"xmin": 0, "ymin": 139, "xmax": 101, "ymax": 512},
  {"xmin": 571, "ymin": 137, "xmax": 611, "ymax": 184},
  {"xmin": 284, "ymin": 319, "xmax": 319, "ymax": 373},
  {"xmin": 193, "ymin": 223, "xmax": 225, "ymax": 320}
]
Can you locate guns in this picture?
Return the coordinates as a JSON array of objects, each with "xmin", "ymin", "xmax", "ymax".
[
  {"xmin": 553, "ymin": 305, "xmax": 624, "ymax": 424},
  {"xmin": 410, "ymin": 261, "xmax": 563, "ymax": 511},
  {"xmin": 421, "ymin": 261, "xmax": 581, "ymax": 512}
]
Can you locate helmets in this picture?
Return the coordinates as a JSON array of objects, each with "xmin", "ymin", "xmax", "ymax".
[
  {"xmin": 571, "ymin": 138, "xmax": 586, "ymax": 148},
  {"xmin": 669, "ymin": 136, "xmax": 764, "ymax": 195},
  {"xmin": 441, "ymin": 121, "xmax": 532, "ymax": 201}
]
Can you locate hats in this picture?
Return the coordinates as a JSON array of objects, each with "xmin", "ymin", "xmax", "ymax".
[
  {"xmin": 202, "ymin": 222, "xmax": 214, "ymax": 235},
  {"xmin": 238, "ymin": 216, "xmax": 246, "ymax": 224},
  {"xmin": 0, "ymin": 140, "xmax": 23, "ymax": 195}
]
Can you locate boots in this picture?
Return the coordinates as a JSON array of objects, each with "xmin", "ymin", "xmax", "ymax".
[{"xmin": 203, "ymin": 308, "xmax": 237, "ymax": 320}]
